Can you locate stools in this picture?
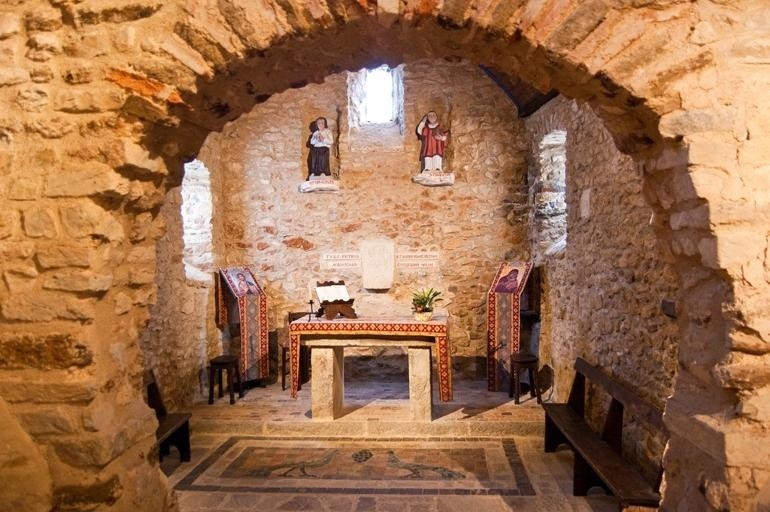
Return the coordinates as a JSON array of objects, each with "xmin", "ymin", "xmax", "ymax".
[
  {"xmin": 509, "ymin": 354, "xmax": 543, "ymax": 404},
  {"xmin": 208, "ymin": 355, "xmax": 244, "ymax": 405}
]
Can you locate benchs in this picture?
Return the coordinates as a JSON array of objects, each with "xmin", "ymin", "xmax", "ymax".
[
  {"xmin": 146, "ymin": 369, "xmax": 191, "ymax": 462},
  {"xmin": 542, "ymin": 357, "xmax": 663, "ymax": 512}
]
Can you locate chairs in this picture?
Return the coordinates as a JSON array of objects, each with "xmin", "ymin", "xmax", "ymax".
[{"xmin": 281, "ymin": 313, "xmax": 311, "ymax": 391}]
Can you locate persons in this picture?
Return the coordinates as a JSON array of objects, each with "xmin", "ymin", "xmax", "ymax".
[
  {"xmin": 417, "ymin": 110, "xmax": 450, "ymax": 172},
  {"xmin": 305, "ymin": 118, "xmax": 334, "ymax": 177},
  {"xmin": 495, "ymin": 268, "xmax": 519, "ymax": 291},
  {"xmin": 236, "ymin": 272, "xmax": 258, "ymax": 294},
  {"xmin": 508, "ymin": 273, "xmax": 518, "ymax": 288}
]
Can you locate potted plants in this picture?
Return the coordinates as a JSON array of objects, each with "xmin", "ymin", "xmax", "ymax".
[{"xmin": 411, "ymin": 288, "xmax": 443, "ymax": 321}]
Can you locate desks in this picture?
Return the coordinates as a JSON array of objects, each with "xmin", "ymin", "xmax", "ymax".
[{"xmin": 289, "ymin": 314, "xmax": 453, "ymax": 421}]
